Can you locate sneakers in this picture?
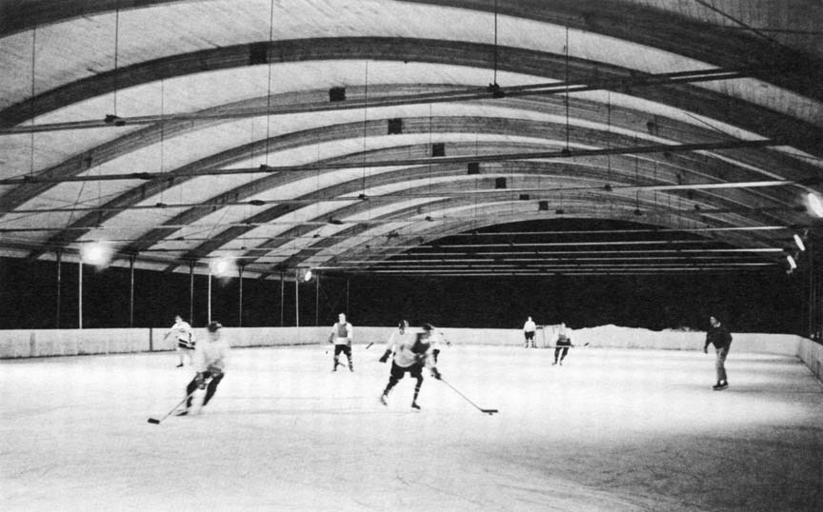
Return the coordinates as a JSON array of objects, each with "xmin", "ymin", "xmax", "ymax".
[
  {"xmin": 712, "ymin": 380, "xmax": 723, "ymax": 388},
  {"xmin": 380, "ymin": 396, "xmax": 389, "ymax": 406},
  {"xmin": 176, "ymin": 408, "xmax": 186, "ymax": 416},
  {"xmin": 412, "ymin": 402, "xmax": 421, "ymax": 409},
  {"xmin": 720, "ymin": 382, "xmax": 727, "ymax": 387}
]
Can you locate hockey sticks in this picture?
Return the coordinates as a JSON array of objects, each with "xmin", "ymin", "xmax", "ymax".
[
  {"xmin": 432, "ymin": 373, "xmax": 498, "ymax": 413},
  {"xmin": 546, "ymin": 343, "xmax": 588, "ymax": 347},
  {"xmin": 149, "ymin": 375, "xmax": 213, "ymax": 424},
  {"xmin": 332, "ymin": 342, "xmax": 374, "ymax": 351}
]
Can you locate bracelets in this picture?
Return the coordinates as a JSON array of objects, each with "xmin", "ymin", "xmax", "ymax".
[{"xmin": 446, "ymin": 340, "xmax": 450, "ymax": 345}]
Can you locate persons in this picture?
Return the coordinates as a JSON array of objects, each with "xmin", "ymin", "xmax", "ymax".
[
  {"xmin": 381, "ymin": 318, "xmax": 424, "ymax": 411},
  {"xmin": 422, "ymin": 323, "xmax": 453, "ymax": 381},
  {"xmin": 174, "ymin": 321, "xmax": 231, "ymax": 417},
  {"xmin": 703, "ymin": 314, "xmax": 732, "ymax": 388},
  {"xmin": 551, "ymin": 322, "xmax": 571, "ymax": 366},
  {"xmin": 160, "ymin": 311, "xmax": 199, "ymax": 368},
  {"xmin": 409, "ymin": 332, "xmax": 431, "ymax": 366},
  {"xmin": 328, "ymin": 312, "xmax": 355, "ymax": 373},
  {"xmin": 522, "ymin": 316, "xmax": 538, "ymax": 349}
]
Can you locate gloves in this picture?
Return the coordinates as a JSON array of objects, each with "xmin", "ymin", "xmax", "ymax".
[
  {"xmin": 719, "ymin": 348, "xmax": 725, "ymax": 356},
  {"xmin": 432, "ymin": 349, "xmax": 440, "ymax": 364},
  {"xmin": 704, "ymin": 346, "xmax": 708, "ymax": 353},
  {"xmin": 433, "ymin": 367, "xmax": 442, "ymax": 380},
  {"xmin": 196, "ymin": 376, "xmax": 206, "ymax": 389},
  {"xmin": 378, "ymin": 349, "xmax": 393, "ymax": 363},
  {"xmin": 209, "ymin": 367, "xmax": 221, "ymax": 380}
]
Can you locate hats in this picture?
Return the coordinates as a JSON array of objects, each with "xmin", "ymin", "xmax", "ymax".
[
  {"xmin": 399, "ymin": 320, "xmax": 409, "ymax": 329},
  {"xmin": 208, "ymin": 321, "xmax": 222, "ymax": 332},
  {"xmin": 422, "ymin": 323, "xmax": 435, "ymax": 329}
]
